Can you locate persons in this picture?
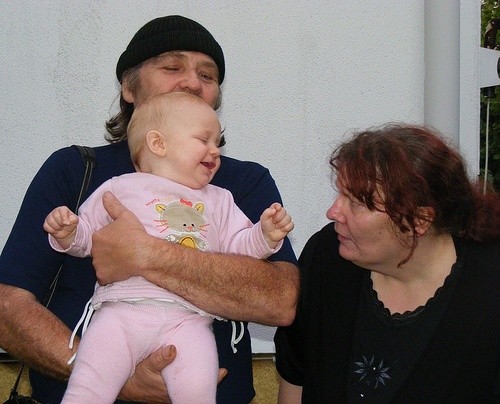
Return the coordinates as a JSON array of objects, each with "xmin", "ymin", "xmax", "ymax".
[
  {"xmin": 1, "ymin": 15, "xmax": 301, "ymax": 403},
  {"xmin": 271, "ymin": 122, "xmax": 500, "ymax": 403},
  {"xmin": 43, "ymin": 93, "xmax": 294, "ymax": 404}
]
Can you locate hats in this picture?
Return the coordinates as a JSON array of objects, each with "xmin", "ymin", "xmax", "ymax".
[{"xmin": 116, "ymin": 15, "xmax": 225, "ymax": 85}]
